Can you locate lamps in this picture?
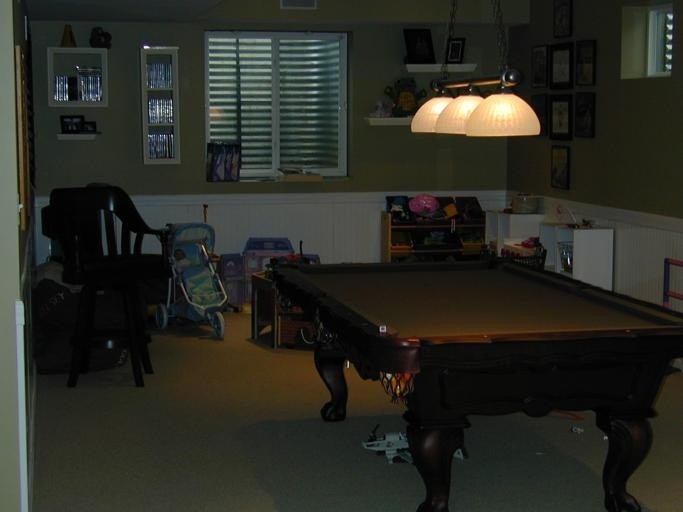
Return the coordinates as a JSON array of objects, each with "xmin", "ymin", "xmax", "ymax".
[{"xmin": 409, "ymin": 0, "xmax": 541, "ymax": 139}]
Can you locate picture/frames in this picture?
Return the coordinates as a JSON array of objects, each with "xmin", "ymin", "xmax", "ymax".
[
  {"xmin": 401, "ymin": 27, "xmax": 436, "ymax": 64},
  {"xmin": 444, "ymin": 36, "xmax": 466, "ymax": 64},
  {"xmin": 529, "ymin": 0, "xmax": 599, "ymax": 192}
]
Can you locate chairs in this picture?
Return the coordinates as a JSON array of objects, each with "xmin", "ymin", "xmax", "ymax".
[{"xmin": 39, "ymin": 181, "xmax": 187, "ymax": 389}]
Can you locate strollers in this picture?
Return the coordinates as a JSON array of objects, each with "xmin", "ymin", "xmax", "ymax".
[{"xmin": 152, "ymin": 222, "xmax": 228, "ymax": 341}]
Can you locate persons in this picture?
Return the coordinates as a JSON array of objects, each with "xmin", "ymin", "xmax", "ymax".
[{"xmin": 276, "ymin": 167, "xmax": 323, "ymax": 184}]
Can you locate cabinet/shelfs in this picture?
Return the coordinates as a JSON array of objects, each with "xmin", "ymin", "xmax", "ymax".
[
  {"xmin": 492, "ymin": 208, "xmax": 545, "ymax": 258},
  {"xmin": 141, "ymin": 47, "xmax": 181, "ymax": 166},
  {"xmin": 539, "ymin": 221, "xmax": 615, "ymax": 293},
  {"xmin": 381, "ymin": 211, "xmax": 486, "ymax": 262}
]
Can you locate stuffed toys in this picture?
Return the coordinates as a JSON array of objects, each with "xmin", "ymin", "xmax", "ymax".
[{"xmin": 384, "ymin": 77, "xmax": 427, "ymax": 117}]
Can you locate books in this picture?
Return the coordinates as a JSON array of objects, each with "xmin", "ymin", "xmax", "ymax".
[
  {"xmin": 144, "ymin": 62, "xmax": 174, "ymax": 161},
  {"xmin": 52, "ymin": 66, "xmax": 102, "ymax": 104}
]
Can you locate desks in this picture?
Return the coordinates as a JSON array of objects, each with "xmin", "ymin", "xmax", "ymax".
[{"xmin": 270, "ymin": 252, "xmax": 682, "ymax": 512}]
